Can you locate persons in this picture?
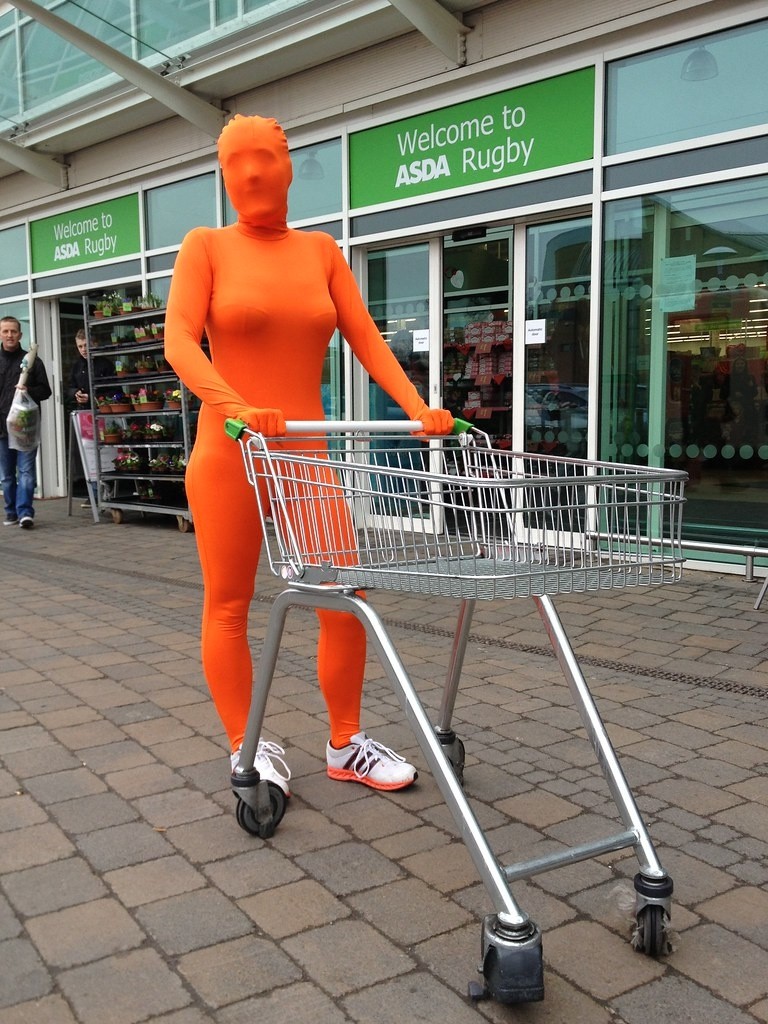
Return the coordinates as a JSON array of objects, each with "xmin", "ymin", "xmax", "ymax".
[
  {"xmin": 719, "ymin": 357, "xmax": 758, "ymax": 471},
  {"xmin": 68, "ymin": 330, "xmax": 122, "ymax": 509},
  {"xmin": 689, "ymin": 366, "xmax": 713, "ymax": 418},
  {"xmin": 0, "ymin": 315, "xmax": 52, "ymax": 526},
  {"xmin": 165, "ymin": 114, "xmax": 455, "ymax": 798}
]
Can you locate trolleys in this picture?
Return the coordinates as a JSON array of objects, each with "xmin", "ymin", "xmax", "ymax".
[{"xmin": 220, "ymin": 416, "xmax": 675, "ymax": 1003}]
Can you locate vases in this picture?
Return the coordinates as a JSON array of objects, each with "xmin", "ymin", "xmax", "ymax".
[
  {"xmin": 152, "ymin": 332, "xmax": 164, "ymax": 340},
  {"xmin": 141, "ymin": 401, "xmax": 161, "ymax": 412},
  {"xmin": 166, "ymin": 400, "xmax": 193, "ymax": 411},
  {"xmin": 92, "ymin": 310, "xmax": 109, "ymax": 319},
  {"xmin": 132, "ymin": 402, "xmax": 142, "ymax": 412},
  {"xmin": 130, "ymin": 435, "xmax": 162, "ymax": 441},
  {"xmin": 118, "ymin": 307, "xmax": 141, "ymax": 316},
  {"xmin": 97, "ymin": 404, "xmax": 112, "ymax": 415},
  {"xmin": 136, "ymin": 335, "xmax": 154, "ymax": 344},
  {"xmin": 110, "ymin": 403, "xmax": 131, "ymax": 413}
]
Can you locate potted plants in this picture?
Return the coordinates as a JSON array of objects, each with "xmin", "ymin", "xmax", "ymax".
[
  {"xmin": 104, "ymin": 420, "xmax": 121, "ymax": 444},
  {"xmin": 140, "ymin": 293, "xmax": 163, "ymax": 312},
  {"xmin": 137, "ymin": 485, "xmax": 162, "ymax": 500},
  {"xmin": 113, "ymin": 359, "xmax": 173, "ymax": 378},
  {"xmin": 12, "ymin": 410, "xmax": 38, "ymax": 449}
]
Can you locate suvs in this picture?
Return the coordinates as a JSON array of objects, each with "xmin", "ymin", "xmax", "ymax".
[{"xmin": 528, "ymin": 382, "xmax": 619, "ymax": 418}]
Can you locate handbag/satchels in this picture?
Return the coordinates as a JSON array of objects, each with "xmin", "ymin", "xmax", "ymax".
[{"xmin": 6, "ymin": 388, "xmax": 41, "ymax": 452}]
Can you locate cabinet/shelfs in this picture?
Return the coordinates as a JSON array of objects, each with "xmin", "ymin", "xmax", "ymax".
[{"xmin": 82, "ymin": 292, "xmax": 201, "ymax": 534}]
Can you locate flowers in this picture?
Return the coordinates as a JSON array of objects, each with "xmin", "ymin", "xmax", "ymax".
[
  {"xmin": 111, "ymin": 447, "xmax": 188, "ymax": 468},
  {"xmin": 128, "ymin": 418, "xmax": 165, "ymax": 432},
  {"xmin": 101, "ymin": 289, "xmax": 134, "ymax": 302},
  {"xmin": 96, "ymin": 387, "xmax": 197, "ymax": 400}
]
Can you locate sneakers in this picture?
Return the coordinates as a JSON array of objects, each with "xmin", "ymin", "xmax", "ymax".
[
  {"xmin": 3, "ymin": 512, "xmax": 19, "ymax": 525},
  {"xmin": 81, "ymin": 497, "xmax": 98, "ymax": 508},
  {"xmin": 229, "ymin": 737, "xmax": 292, "ymax": 798},
  {"xmin": 19, "ymin": 516, "xmax": 34, "ymax": 528},
  {"xmin": 326, "ymin": 729, "xmax": 418, "ymax": 791}
]
